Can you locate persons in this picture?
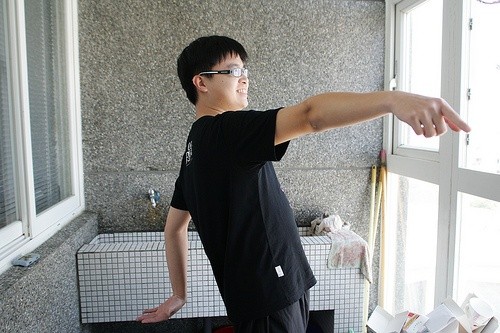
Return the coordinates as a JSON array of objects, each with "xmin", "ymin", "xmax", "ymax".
[{"xmin": 136, "ymin": 35, "xmax": 471, "ymax": 333}]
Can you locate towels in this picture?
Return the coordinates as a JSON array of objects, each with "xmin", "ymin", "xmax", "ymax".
[{"xmin": 311, "ymin": 213, "xmax": 373, "ymax": 284}]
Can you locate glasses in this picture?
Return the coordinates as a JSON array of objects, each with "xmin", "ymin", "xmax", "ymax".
[{"xmin": 198, "ymin": 67, "xmax": 249, "ymax": 77}]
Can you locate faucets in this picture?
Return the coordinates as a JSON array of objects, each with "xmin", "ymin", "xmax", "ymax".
[{"xmin": 147, "ymin": 190, "xmax": 161, "ymax": 208}]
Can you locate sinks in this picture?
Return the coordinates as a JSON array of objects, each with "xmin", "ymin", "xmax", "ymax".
[{"xmin": 77, "ymin": 224, "xmax": 370, "ymax": 324}]
